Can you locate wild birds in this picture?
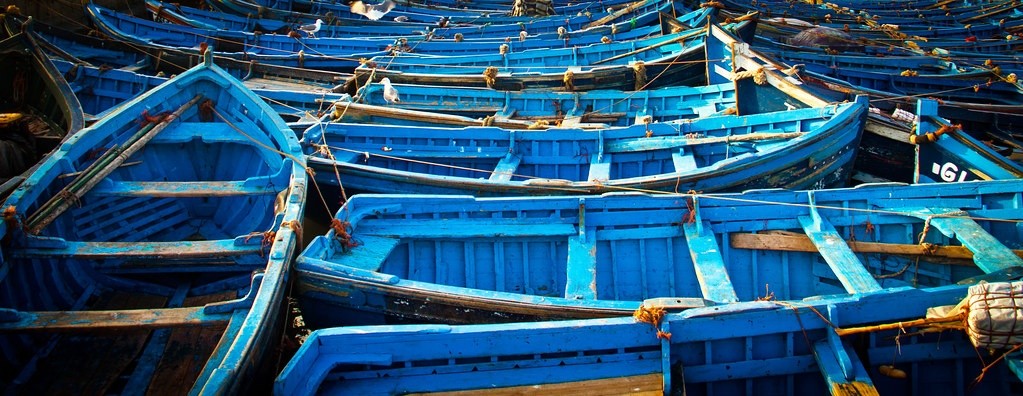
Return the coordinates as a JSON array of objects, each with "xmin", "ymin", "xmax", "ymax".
[
  {"xmin": 394, "ymin": 15, "xmax": 408, "ymax": 23},
  {"xmin": 297, "ymin": 19, "xmax": 326, "ymax": 39},
  {"xmin": 380, "ymin": 77, "xmax": 401, "ymax": 109}
]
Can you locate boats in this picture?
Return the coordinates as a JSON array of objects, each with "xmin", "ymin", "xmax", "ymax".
[
  {"xmin": 0, "ymin": 0, "xmax": 1023, "ymax": 396},
  {"xmin": 1, "ymin": 44, "xmax": 310, "ymax": 395}
]
